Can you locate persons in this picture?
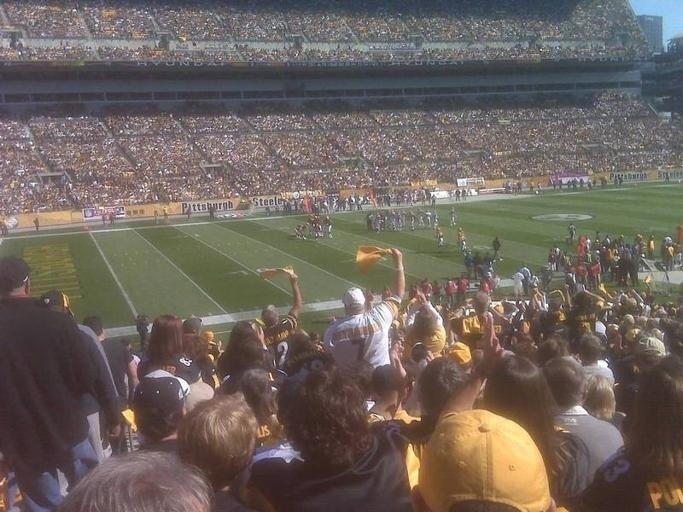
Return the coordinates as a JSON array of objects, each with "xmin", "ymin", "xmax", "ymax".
[{"xmin": 0, "ymin": 0, "xmax": 683, "ymax": 512}]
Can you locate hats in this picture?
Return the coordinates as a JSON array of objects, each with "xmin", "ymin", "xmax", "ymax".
[
  {"xmin": 182, "ymin": 318, "xmax": 202, "ymax": 334},
  {"xmin": 632, "ymin": 335, "xmax": 666, "ymax": 363},
  {"xmin": 40, "ymin": 288, "xmax": 75, "ymax": 319},
  {"xmin": 420, "ymin": 409, "xmax": 552, "ymax": 511},
  {"xmin": 0, "ymin": 256, "xmax": 32, "ymax": 287},
  {"xmin": 261, "ymin": 304, "xmax": 278, "ymax": 325},
  {"xmin": 342, "ymin": 286, "xmax": 366, "ymax": 308},
  {"xmin": 134, "ymin": 367, "xmax": 190, "ymax": 419}
]
